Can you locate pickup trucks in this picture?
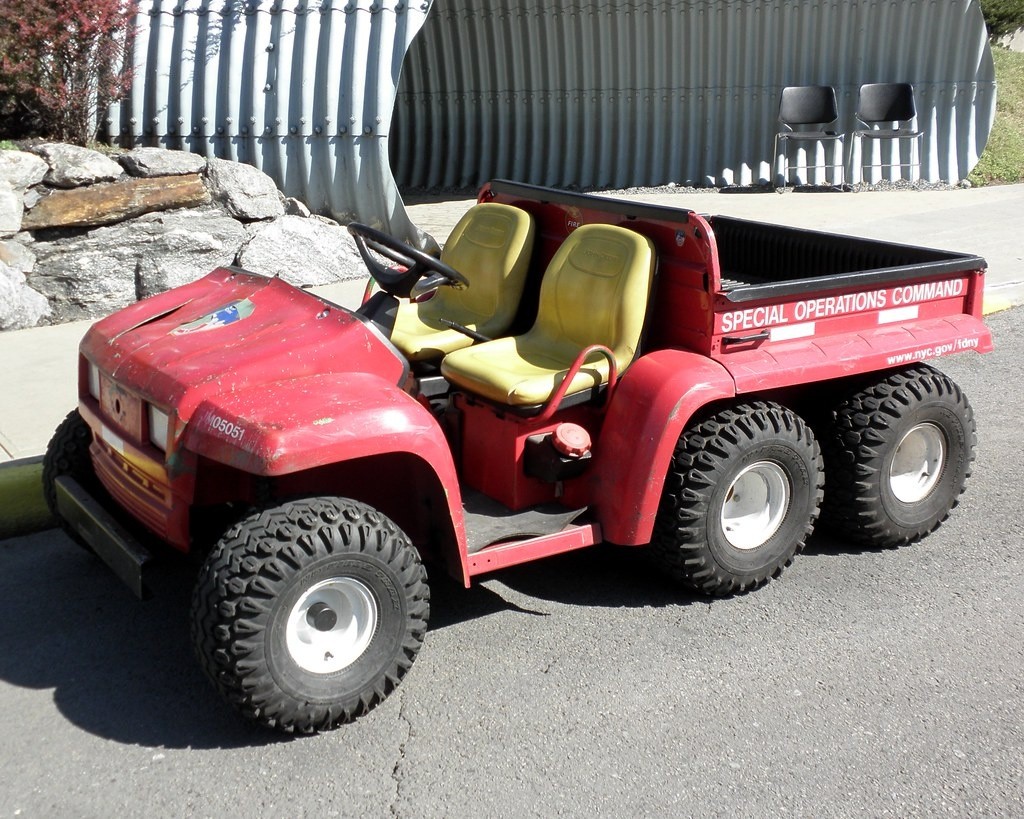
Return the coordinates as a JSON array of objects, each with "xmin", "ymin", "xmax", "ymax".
[{"xmin": 42, "ymin": 175, "xmax": 993, "ymax": 740}]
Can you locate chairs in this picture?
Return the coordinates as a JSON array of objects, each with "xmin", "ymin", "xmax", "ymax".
[
  {"xmin": 771, "ymin": 86, "xmax": 844, "ymax": 195},
  {"xmin": 439, "ymin": 224, "xmax": 662, "ymax": 413},
  {"xmin": 393, "ymin": 201, "xmax": 535, "ymax": 360},
  {"xmin": 846, "ymin": 82, "xmax": 924, "ymax": 193}
]
